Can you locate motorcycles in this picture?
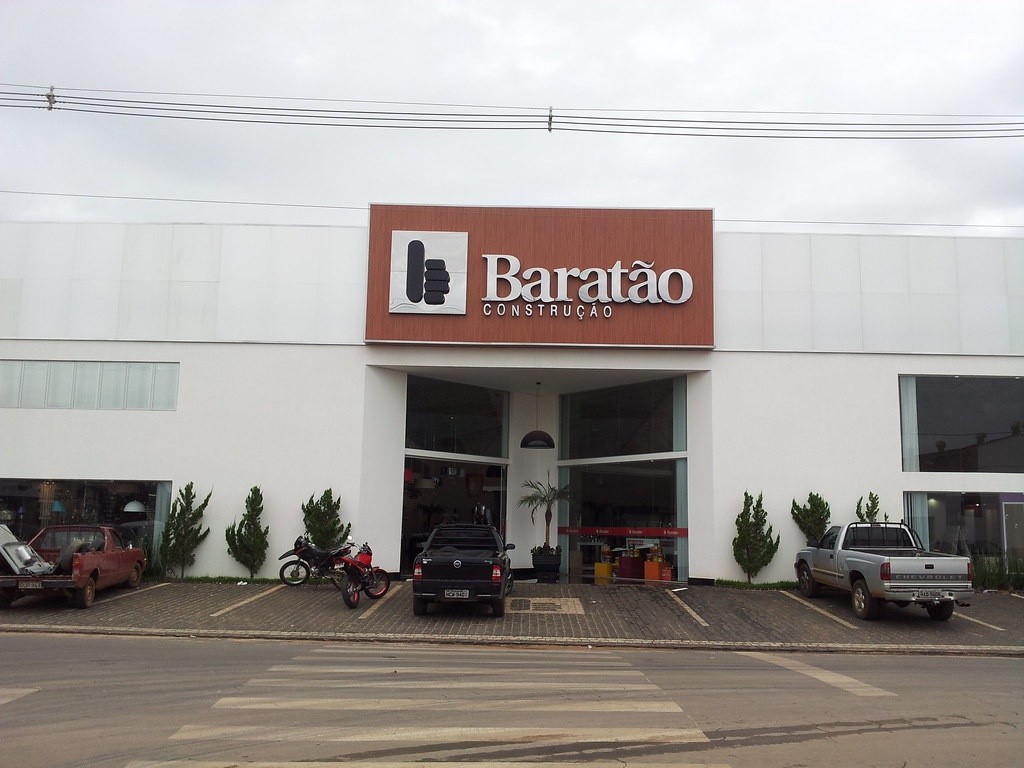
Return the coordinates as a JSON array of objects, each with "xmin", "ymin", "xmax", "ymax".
[
  {"xmin": 331, "ymin": 535, "xmax": 390, "ymax": 609},
  {"xmin": 279, "ymin": 527, "xmax": 354, "ymax": 590}
]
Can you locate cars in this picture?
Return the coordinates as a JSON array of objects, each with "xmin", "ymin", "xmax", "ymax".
[{"xmin": 931, "ymin": 538, "xmax": 1022, "ymax": 572}]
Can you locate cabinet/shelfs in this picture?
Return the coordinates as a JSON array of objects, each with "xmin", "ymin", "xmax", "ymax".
[
  {"xmin": 644, "ymin": 562, "xmax": 670, "ymax": 580},
  {"xmin": 577, "ymin": 542, "xmax": 602, "ymax": 570},
  {"xmin": 662, "ymin": 570, "xmax": 672, "ymax": 581},
  {"xmin": 612, "ymin": 565, "xmax": 619, "ymax": 576},
  {"xmin": 619, "ymin": 557, "xmax": 642, "ymax": 577},
  {"xmin": 595, "ymin": 563, "xmax": 612, "ymax": 577}
]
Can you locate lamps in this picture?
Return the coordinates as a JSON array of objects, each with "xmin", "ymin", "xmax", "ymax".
[
  {"xmin": 124, "ymin": 500, "xmax": 146, "ymax": 512},
  {"xmin": 520, "ymin": 382, "xmax": 555, "ymax": 448}
]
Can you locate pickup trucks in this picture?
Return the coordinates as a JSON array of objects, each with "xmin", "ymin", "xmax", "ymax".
[
  {"xmin": 411, "ymin": 522, "xmax": 515, "ymax": 617},
  {"xmin": 0, "ymin": 525, "xmax": 146, "ymax": 609},
  {"xmin": 793, "ymin": 521, "xmax": 974, "ymax": 621}
]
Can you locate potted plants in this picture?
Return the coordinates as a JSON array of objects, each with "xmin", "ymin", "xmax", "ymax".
[{"xmin": 515, "ymin": 469, "xmax": 576, "ymax": 584}]
